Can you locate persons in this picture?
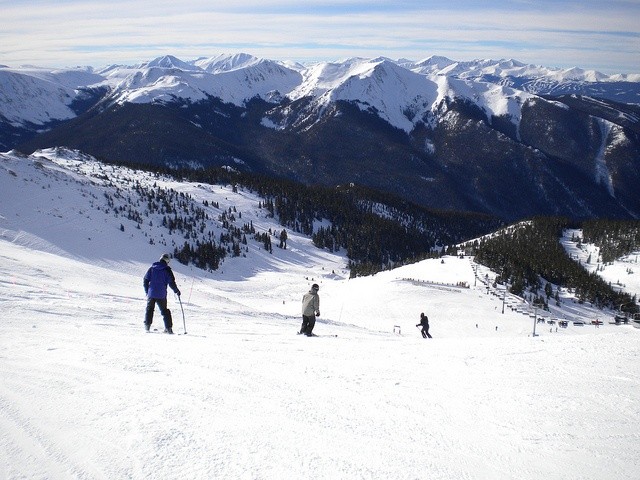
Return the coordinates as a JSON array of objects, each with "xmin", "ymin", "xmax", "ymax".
[
  {"xmin": 415, "ymin": 312, "xmax": 432, "ymax": 338},
  {"xmin": 300, "ymin": 284, "xmax": 320, "ymax": 337},
  {"xmin": 143, "ymin": 253, "xmax": 181, "ymax": 335}
]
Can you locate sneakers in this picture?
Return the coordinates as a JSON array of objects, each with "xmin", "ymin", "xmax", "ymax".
[
  {"xmin": 145, "ymin": 324, "xmax": 151, "ymax": 330},
  {"xmin": 163, "ymin": 328, "xmax": 173, "ymax": 334}
]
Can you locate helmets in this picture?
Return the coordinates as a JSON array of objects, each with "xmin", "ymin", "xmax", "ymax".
[
  {"xmin": 311, "ymin": 283, "xmax": 318, "ymax": 291},
  {"xmin": 159, "ymin": 254, "xmax": 171, "ymax": 263}
]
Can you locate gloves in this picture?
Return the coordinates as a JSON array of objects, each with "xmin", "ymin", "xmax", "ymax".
[
  {"xmin": 316, "ymin": 313, "xmax": 320, "ymax": 316},
  {"xmin": 177, "ymin": 290, "xmax": 181, "ymax": 297}
]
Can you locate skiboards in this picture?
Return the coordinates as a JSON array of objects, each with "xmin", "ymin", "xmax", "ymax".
[
  {"xmin": 146, "ymin": 331, "xmax": 207, "ymax": 337},
  {"xmin": 297, "ymin": 332, "xmax": 338, "ymax": 337}
]
